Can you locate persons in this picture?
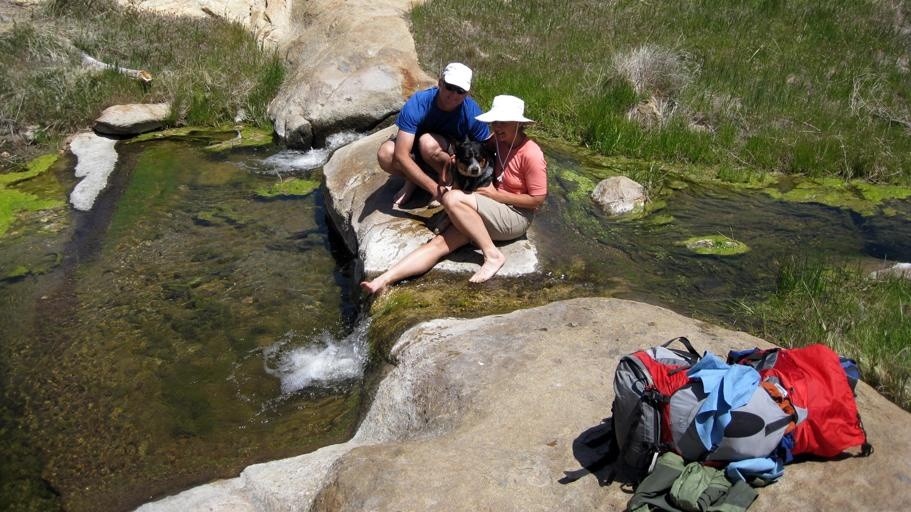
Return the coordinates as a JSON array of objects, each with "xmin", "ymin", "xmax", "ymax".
[
  {"xmin": 359, "ymin": 94, "xmax": 547, "ymax": 295},
  {"xmin": 377, "ymin": 61, "xmax": 489, "ymax": 207}
]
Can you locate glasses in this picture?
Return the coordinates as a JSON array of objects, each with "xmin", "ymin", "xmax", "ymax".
[{"xmin": 442, "ymin": 79, "xmax": 466, "ymax": 95}]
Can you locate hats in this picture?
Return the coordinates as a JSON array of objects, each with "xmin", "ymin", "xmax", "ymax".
[
  {"xmin": 473, "ymin": 93, "xmax": 536, "ymax": 126},
  {"xmin": 442, "ymin": 62, "xmax": 474, "ymax": 92}
]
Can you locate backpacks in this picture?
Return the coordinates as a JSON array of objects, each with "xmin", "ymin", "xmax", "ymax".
[{"xmin": 610, "ymin": 333, "xmax": 876, "ymax": 474}]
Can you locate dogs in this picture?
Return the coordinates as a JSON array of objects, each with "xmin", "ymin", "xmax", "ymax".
[{"xmin": 448, "ymin": 135, "xmax": 500, "ymax": 195}]
